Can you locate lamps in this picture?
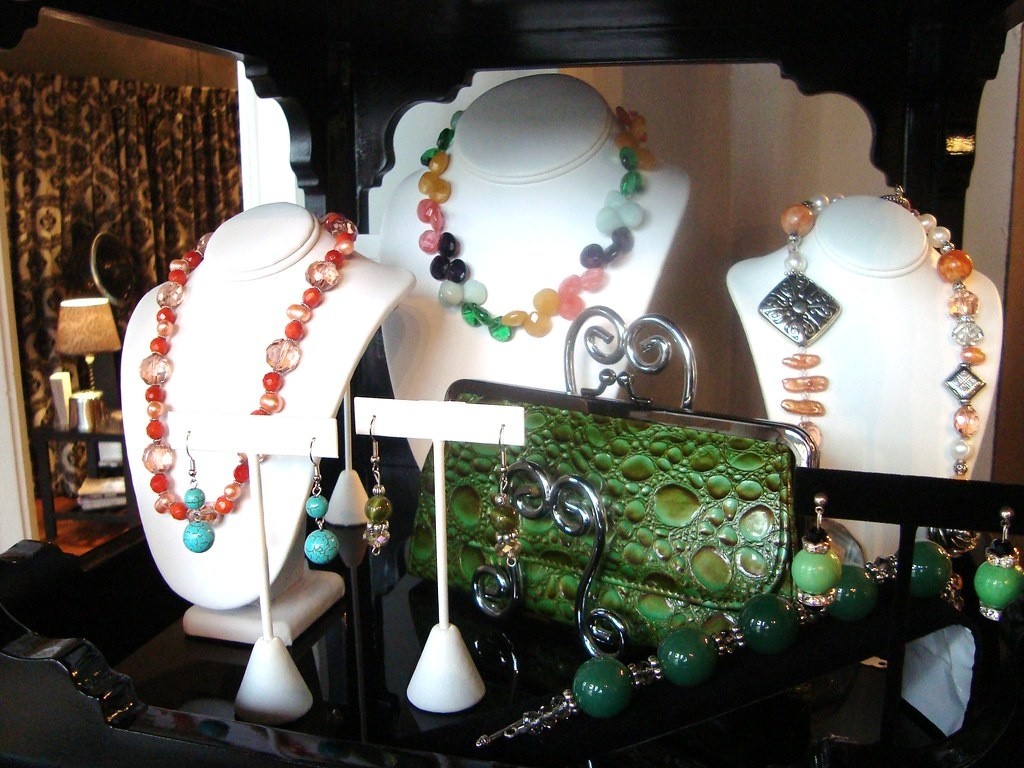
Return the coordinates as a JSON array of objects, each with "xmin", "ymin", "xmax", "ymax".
[{"xmin": 55, "ymin": 298, "xmax": 121, "ymax": 390}]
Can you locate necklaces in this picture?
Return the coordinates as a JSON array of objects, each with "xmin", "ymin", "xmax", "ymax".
[
  {"xmin": 756, "ymin": 189, "xmax": 987, "ymax": 477},
  {"xmin": 138, "ymin": 212, "xmax": 356, "ymax": 550},
  {"xmin": 413, "ymin": 102, "xmax": 651, "ymax": 340}
]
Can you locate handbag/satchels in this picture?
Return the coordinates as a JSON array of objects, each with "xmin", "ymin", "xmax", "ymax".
[{"xmin": 406, "ymin": 305, "xmax": 821, "ymax": 654}]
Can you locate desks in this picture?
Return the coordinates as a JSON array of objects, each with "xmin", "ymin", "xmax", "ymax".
[{"xmin": 34, "ymin": 426, "xmax": 139, "ymax": 538}]
